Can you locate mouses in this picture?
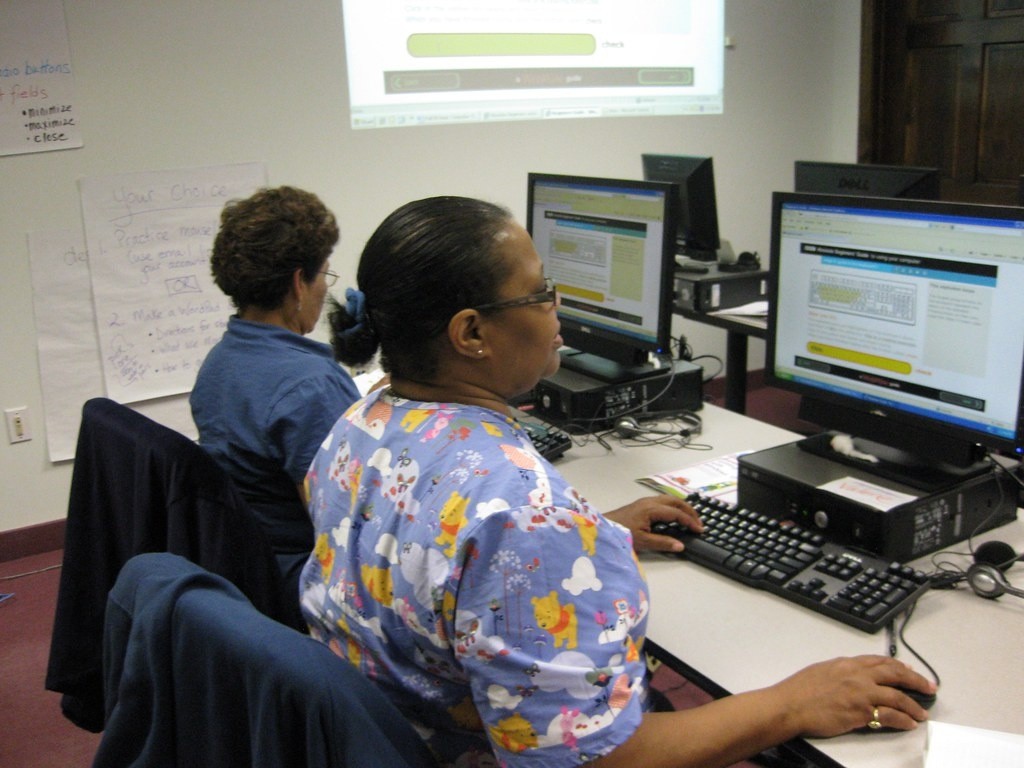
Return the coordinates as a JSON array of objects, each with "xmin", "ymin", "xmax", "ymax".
[{"xmin": 854, "ymin": 682, "xmax": 936, "ymax": 735}]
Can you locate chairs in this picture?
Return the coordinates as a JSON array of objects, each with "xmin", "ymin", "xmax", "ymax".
[
  {"xmin": 46, "ymin": 397, "xmax": 281, "ymax": 734},
  {"xmin": 87, "ymin": 554, "xmax": 435, "ymax": 768}
]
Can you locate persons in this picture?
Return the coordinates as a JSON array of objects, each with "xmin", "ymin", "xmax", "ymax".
[
  {"xmin": 192, "ymin": 185, "xmax": 361, "ymax": 585},
  {"xmin": 301, "ymin": 193, "xmax": 939, "ymax": 768}
]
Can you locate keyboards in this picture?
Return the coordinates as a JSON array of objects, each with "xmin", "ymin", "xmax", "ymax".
[
  {"xmin": 519, "ymin": 421, "xmax": 572, "ymax": 461},
  {"xmin": 649, "ymin": 491, "xmax": 931, "ymax": 635}
]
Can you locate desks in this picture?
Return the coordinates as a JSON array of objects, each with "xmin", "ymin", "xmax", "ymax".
[
  {"xmin": 670, "ymin": 303, "xmax": 768, "ymax": 414},
  {"xmin": 357, "ymin": 372, "xmax": 1024, "ymax": 767}
]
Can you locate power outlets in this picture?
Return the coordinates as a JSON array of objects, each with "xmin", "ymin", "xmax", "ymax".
[{"xmin": 5, "ymin": 404, "xmax": 35, "ymax": 445}]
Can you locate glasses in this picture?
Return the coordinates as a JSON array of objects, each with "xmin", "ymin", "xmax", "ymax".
[
  {"xmin": 474, "ymin": 277, "xmax": 560, "ymax": 312},
  {"xmin": 316, "ymin": 269, "xmax": 339, "ymax": 288}
]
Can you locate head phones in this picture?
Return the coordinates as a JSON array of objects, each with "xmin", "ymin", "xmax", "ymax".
[
  {"xmin": 614, "ymin": 407, "xmax": 703, "ymax": 438},
  {"xmin": 965, "ymin": 541, "xmax": 1024, "ymax": 600},
  {"xmin": 717, "ymin": 251, "xmax": 760, "ymax": 272}
]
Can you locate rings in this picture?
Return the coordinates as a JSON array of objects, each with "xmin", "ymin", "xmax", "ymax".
[{"xmin": 867, "ymin": 709, "xmax": 882, "ymax": 729}]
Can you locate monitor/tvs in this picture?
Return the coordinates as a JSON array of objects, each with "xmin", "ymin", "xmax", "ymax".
[
  {"xmin": 764, "ymin": 160, "xmax": 1024, "ymax": 491},
  {"xmin": 526, "ymin": 152, "xmax": 721, "ymax": 386}
]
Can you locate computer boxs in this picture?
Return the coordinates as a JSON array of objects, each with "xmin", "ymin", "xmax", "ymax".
[
  {"xmin": 672, "ymin": 262, "xmax": 769, "ymax": 314},
  {"xmin": 736, "ymin": 438, "xmax": 1022, "ymax": 563},
  {"xmin": 505, "ymin": 350, "xmax": 704, "ymax": 435}
]
